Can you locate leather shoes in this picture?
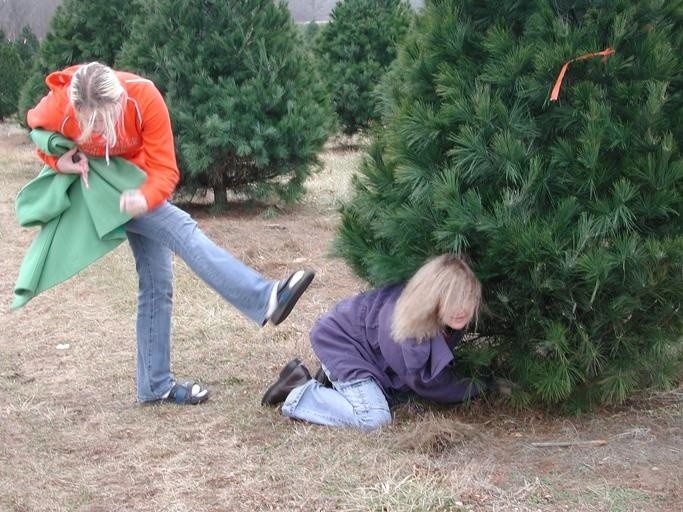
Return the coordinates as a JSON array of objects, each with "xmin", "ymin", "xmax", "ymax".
[
  {"xmin": 313, "ymin": 369, "xmax": 333, "ymax": 389},
  {"xmin": 260, "ymin": 358, "xmax": 310, "ymax": 406}
]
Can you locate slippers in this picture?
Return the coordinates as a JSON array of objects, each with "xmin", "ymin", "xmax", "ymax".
[
  {"xmin": 270, "ymin": 269, "xmax": 313, "ymax": 325},
  {"xmin": 157, "ymin": 381, "xmax": 209, "ymax": 404}
]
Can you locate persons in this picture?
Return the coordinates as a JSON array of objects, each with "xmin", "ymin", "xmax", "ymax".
[
  {"xmin": 25, "ymin": 61, "xmax": 314, "ymax": 407},
  {"xmin": 260, "ymin": 251, "xmax": 498, "ymax": 434}
]
